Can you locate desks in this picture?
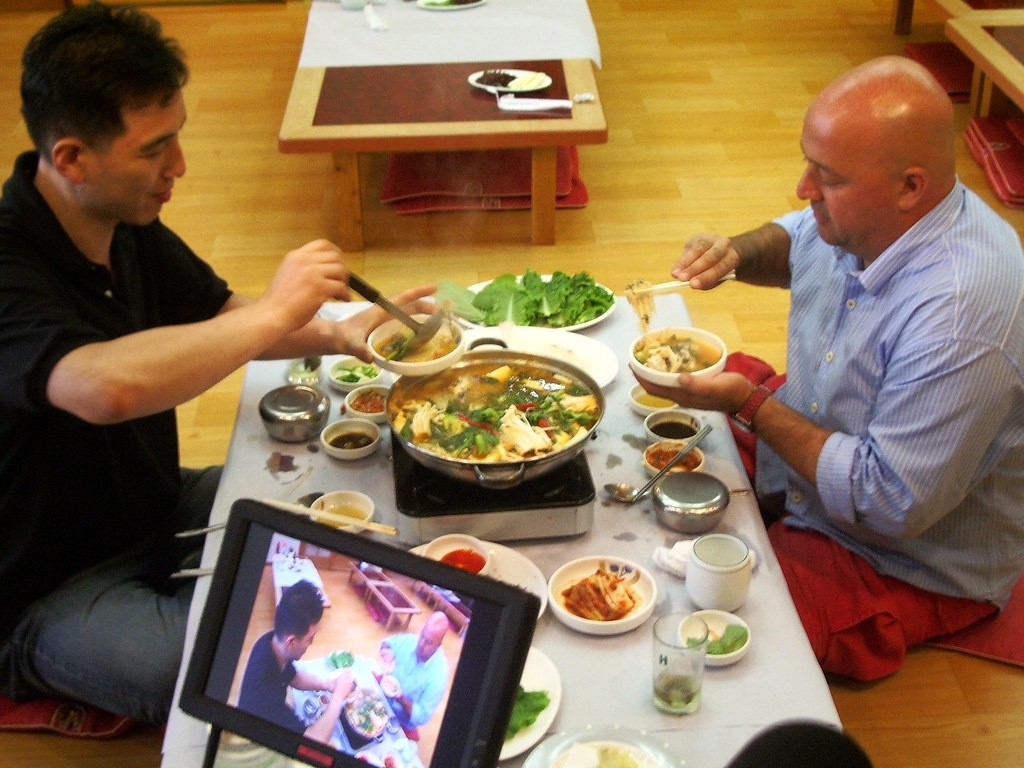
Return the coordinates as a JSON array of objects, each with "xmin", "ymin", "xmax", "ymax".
[
  {"xmin": 437, "ymin": 597, "xmax": 473, "ymax": 638},
  {"xmin": 366, "ymin": 583, "xmax": 424, "ymax": 633},
  {"xmin": 349, "ymin": 558, "xmax": 393, "ymax": 600},
  {"xmin": 292, "ymin": 655, "xmax": 425, "ymax": 768},
  {"xmin": 160, "ymin": 282, "xmax": 844, "ymax": 768},
  {"xmin": 893, "ymin": 0, "xmax": 1024, "ymax": 116},
  {"xmin": 271, "ymin": 552, "xmax": 332, "ymax": 614},
  {"xmin": 418, "ymin": 582, "xmax": 457, "ymax": 610},
  {"xmin": 278, "ymin": 0, "xmax": 609, "ymax": 246}
]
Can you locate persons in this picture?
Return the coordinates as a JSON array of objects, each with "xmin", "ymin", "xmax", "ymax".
[
  {"xmin": 376, "ymin": 610, "xmax": 450, "ymax": 727},
  {"xmin": 728, "ymin": 721, "xmax": 875, "ymax": 768},
  {"xmin": 0, "ymin": 4, "xmax": 445, "ymax": 723},
  {"xmin": 237, "ymin": 579, "xmax": 355, "ymax": 745},
  {"xmin": 630, "ymin": 54, "xmax": 1024, "ymax": 681}
]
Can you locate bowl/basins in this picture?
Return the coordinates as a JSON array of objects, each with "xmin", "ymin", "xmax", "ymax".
[
  {"xmin": 651, "ymin": 472, "xmax": 729, "ymax": 534},
  {"xmin": 309, "ymin": 490, "xmax": 376, "ymax": 535},
  {"xmin": 339, "ymin": 0, "xmax": 368, "ymax": 10},
  {"xmin": 678, "ymin": 609, "xmax": 751, "ymax": 667},
  {"xmin": 344, "ymin": 385, "xmax": 391, "ymax": 424},
  {"xmin": 627, "ymin": 325, "xmax": 728, "ymax": 388},
  {"xmin": 328, "ymin": 356, "xmax": 385, "ymax": 394},
  {"xmin": 547, "ymin": 555, "xmax": 659, "ymax": 636},
  {"xmin": 353, "ymin": 716, "xmax": 425, "ymax": 768},
  {"xmin": 628, "ymin": 383, "xmax": 680, "ymax": 416},
  {"xmin": 258, "ymin": 383, "xmax": 330, "ymax": 443},
  {"xmin": 319, "ymin": 417, "xmax": 382, "ymax": 461},
  {"xmin": 643, "ymin": 409, "xmax": 705, "ymax": 445},
  {"xmin": 366, "ymin": 313, "xmax": 467, "ymax": 377},
  {"xmin": 303, "ymin": 687, "xmax": 331, "ymax": 720},
  {"xmin": 422, "ymin": 533, "xmax": 492, "ymax": 577},
  {"xmin": 380, "ymin": 674, "xmax": 401, "ymax": 698},
  {"xmin": 643, "ymin": 440, "xmax": 706, "ymax": 479}
]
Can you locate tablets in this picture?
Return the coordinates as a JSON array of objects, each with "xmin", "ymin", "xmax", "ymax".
[{"xmin": 177, "ymin": 503, "xmax": 541, "ymax": 768}]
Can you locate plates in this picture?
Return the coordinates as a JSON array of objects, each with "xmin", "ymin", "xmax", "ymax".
[
  {"xmin": 407, "ymin": 540, "xmax": 549, "ymax": 620},
  {"xmin": 450, "ymin": 274, "xmax": 617, "ymax": 332},
  {"xmin": 326, "ymin": 649, "xmax": 355, "ymax": 671},
  {"xmin": 462, "ymin": 326, "xmax": 619, "ymax": 389},
  {"xmin": 415, "ymin": 0, "xmax": 488, "ymax": 12},
  {"xmin": 519, "ymin": 724, "xmax": 680, "ymax": 768},
  {"xmin": 499, "ymin": 645, "xmax": 563, "ymax": 762},
  {"xmin": 467, "ymin": 68, "xmax": 553, "ymax": 92}
]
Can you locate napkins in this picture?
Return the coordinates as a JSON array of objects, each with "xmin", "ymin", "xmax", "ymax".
[
  {"xmin": 392, "ymin": 738, "xmax": 408, "ymax": 750},
  {"xmin": 654, "ymin": 539, "xmax": 756, "ymax": 577}
]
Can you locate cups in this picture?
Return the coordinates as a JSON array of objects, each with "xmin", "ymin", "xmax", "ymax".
[
  {"xmin": 287, "ymin": 311, "xmax": 324, "ymax": 387},
  {"xmin": 685, "ymin": 533, "xmax": 751, "ymax": 613},
  {"xmin": 652, "ymin": 612, "xmax": 708, "ymax": 718},
  {"xmin": 402, "ymin": 739, "xmax": 418, "ymax": 762}
]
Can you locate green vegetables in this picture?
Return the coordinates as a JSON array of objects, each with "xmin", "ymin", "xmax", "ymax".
[
  {"xmin": 435, "ymin": 268, "xmax": 614, "ymax": 327},
  {"xmin": 335, "ymin": 365, "xmax": 375, "ymax": 381},
  {"xmin": 504, "ymin": 686, "xmax": 550, "ymax": 740},
  {"xmin": 685, "ymin": 623, "xmax": 751, "ymax": 656}
]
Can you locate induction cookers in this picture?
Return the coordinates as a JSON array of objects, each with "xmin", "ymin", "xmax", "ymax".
[
  {"xmin": 337, "ymin": 717, "xmax": 386, "ymax": 757},
  {"xmin": 391, "ymin": 428, "xmax": 597, "ymax": 546}
]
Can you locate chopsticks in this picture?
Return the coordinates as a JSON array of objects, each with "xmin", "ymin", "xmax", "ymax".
[
  {"xmin": 630, "ymin": 269, "xmax": 737, "ymax": 294},
  {"xmin": 630, "ymin": 424, "xmax": 714, "ymax": 502},
  {"xmin": 259, "ymin": 497, "xmax": 400, "ymax": 537}
]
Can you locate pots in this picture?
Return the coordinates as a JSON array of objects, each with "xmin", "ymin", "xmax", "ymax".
[
  {"xmin": 344, "ymin": 688, "xmax": 390, "ymax": 744},
  {"xmin": 385, "ymin": 337, "xmax": 607, "ymax": 491}
]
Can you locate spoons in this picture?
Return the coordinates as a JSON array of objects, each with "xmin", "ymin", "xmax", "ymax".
[
  {"xmin": 175, "ymin": 493, "xmax": 325, "ymax": 537},
  {"xmin": 604, "ymin": 483, "xmax": 752, "ymax": 502}
]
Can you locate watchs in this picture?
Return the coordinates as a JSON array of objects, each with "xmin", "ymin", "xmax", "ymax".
[
  {"xmin": 729, "ymin": 384, "xmax": 772, "ymax": 434},
  {"xmin": 396, "ymin": 696, "xmax": 404, "ymax": 701}
]
